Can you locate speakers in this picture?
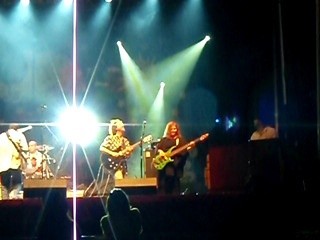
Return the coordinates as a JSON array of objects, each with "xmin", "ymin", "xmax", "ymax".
[
  {"xmin": 24, "ymin": 179, "xmax": 68, "ymax": 198},
  {"xmin": 115, "ymin": 177, "xmax": 158, "ymax": 195}
]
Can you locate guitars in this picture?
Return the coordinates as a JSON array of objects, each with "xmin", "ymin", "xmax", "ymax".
[
  {"xmin": 101, "ymin": 134, "xmax": 152, "ymax": 172},
  {"xmin": 152, "ymin": 133, "xmax": 209, "ymax": 170}
]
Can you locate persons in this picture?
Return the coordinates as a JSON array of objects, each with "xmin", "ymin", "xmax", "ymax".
[
  {"xmin": 0, "ymin": 123, "xmax": 46, "ymax": 199},
  {"xmin": 156, "ymin": 121, "xmax": 194, "ymax": 195},
  {"xmin": 100, "ymin": 188, "xmax": 143, "ymax": 240},
  {"xmin": 84, "ymin": 119, "xmax": 133, "ymax": 198},
  {"xmin": 250, "ymin": 118, "xmax": 278, "ymax": 140},
  {"xmin": 34, "ymin": 187, "xmax": 81, "ymax": 240}
]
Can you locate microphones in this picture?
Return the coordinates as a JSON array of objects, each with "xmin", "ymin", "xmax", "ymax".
[{"xmin": 143, "ymin": 121, "xmax": 146, "ymax": 131}]
